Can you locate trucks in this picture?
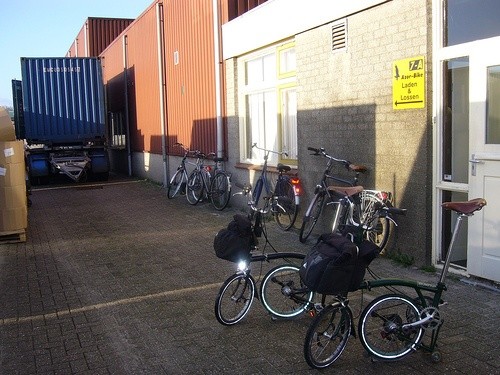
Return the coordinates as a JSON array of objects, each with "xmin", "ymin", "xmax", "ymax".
[{"xmin": 12, "ymin": 55, "xmax": 111, "ymax": 187}]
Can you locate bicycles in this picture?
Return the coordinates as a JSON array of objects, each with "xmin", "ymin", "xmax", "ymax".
[
  {"xmin": 303, "ymin": 188, "xmax": 487, "ymax": 370},
  {"xmin": 215, "ymin": 182, "xmax": 364, "ymax": 326},
  {"xmin": 246, "ymin": 143, "xmax": 301, "ymax": 231},
  {"xmin": 299, "ymin": 146, "xmax": 390, "ymax": 254},
  {"xmin": 185, "ymin": 152, "xmax": 232, "ymax": 211},
  {"xmin": 167, "ymin": 142, "xmax": 212, "ymax": 203}
]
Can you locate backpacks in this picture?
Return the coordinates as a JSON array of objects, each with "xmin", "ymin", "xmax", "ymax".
[
  {"xmin": 299, "ymin": 233, "xmax": 357, "ymax": 295},
  {"xmin": 214, "ymin": 214, "xmax": 251, "ymax": 262}
]
